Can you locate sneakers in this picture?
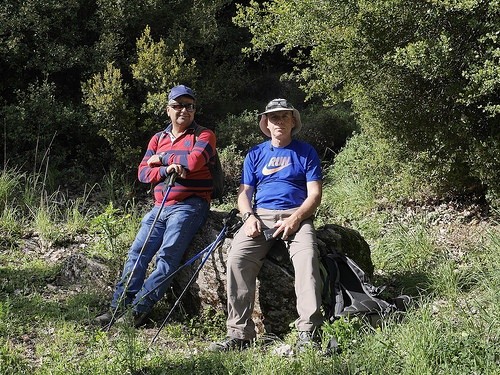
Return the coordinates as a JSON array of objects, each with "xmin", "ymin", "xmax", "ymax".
[
  {"xmin": 95, "ymin": 311, "xmax": 116, "ymax": 324},
  {"xmin": 117, "ymin": 312, "xmax": 144, "ymax": 328}
]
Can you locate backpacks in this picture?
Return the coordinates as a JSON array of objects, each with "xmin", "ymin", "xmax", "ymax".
[
  {"xmin": 154, "ymin": 126, "xmax": 224, "ymax": 204},
  {"xmin": 328, "ymin": 247, "xmax": 413, "ymax": 332}
]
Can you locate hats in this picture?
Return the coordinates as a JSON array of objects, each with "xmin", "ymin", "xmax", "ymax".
[
  {"xmin": 258, "ymin": 98, "xmax": 302, "ymax": 137},
  {"xmin": 169, "ymin": 85, "xmax": 196, "ymax": 101}
]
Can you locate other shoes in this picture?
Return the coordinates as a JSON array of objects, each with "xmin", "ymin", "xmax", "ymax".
[
  {"xmin": 212, "ymin": 337, "xmax": 253, "ymax": 351},
  {"xmin": 298, "ymin": 330, "xmax": 319, "ymax": 348}
]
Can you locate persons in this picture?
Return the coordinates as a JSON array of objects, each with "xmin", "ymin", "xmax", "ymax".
[
  {"xmin": 92, "ymin": 86, "xmax": 217, "ymax": 325},
  {"xmin": 209, "ymin": 99, "xmax": 322, "ymax": 353}
]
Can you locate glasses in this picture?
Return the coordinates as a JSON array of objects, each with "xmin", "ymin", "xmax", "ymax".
[
  {"xmin": 168, "ymin": 104, "xmax": 196, "ymax": 110},
  {"xmin": 267, "ymin": 100, "xmax": 289, "ymax": 109}
]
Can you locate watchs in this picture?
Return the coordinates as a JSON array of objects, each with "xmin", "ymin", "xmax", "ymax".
[{"xmin": 242, "ymin": 212, "xmax": 255, "ymax": 223}]
